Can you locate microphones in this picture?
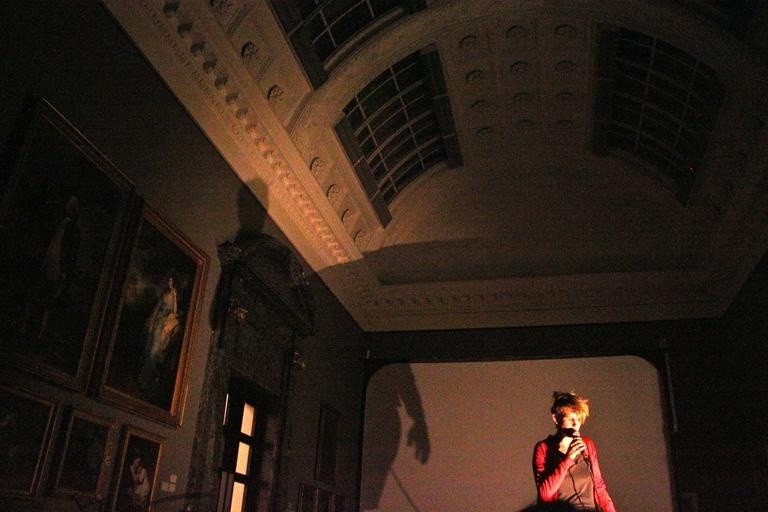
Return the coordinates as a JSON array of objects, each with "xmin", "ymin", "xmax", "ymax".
[{"xmin": 573, "ymin": 430, "xmax": 589, "ymax": 464}]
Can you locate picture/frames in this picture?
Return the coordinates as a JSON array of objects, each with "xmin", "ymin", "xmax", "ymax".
[
  {"xmin": 0, "ymin": 92, "xmax": 139, "ymax": 397},
  {"xmin": 0, "ymin": 381, "xmax": 65, "ymax": 500},
  {"xmin": 46, "ymin": 403, "xmax": 119, "ymax": 504},
  {"xmin": 107, "ymin": 418, "xmax": 167, "ymax": 512},
  {"xmin": 89, "ymin": 188, "xmax": 211, "ymax": 432}
]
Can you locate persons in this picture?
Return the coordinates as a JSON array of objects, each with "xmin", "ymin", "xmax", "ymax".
[
  {"xmin": 532, "ymin": 389, "xmax": 618, "ymax": 511},
  {"xmin": 145, "ymin": 274, "xmax": 178, "ymax": 360},
  {"xmin": 212, "ymin": 180, "xmax": 497, "ymax": 505},
  {"xmin": 21, "ymin": 195, "xmax": 82, "ymax": 344},
  {"xmin": 127, "ymin": 449, "xmax": 150, "ymax": 505}
]
